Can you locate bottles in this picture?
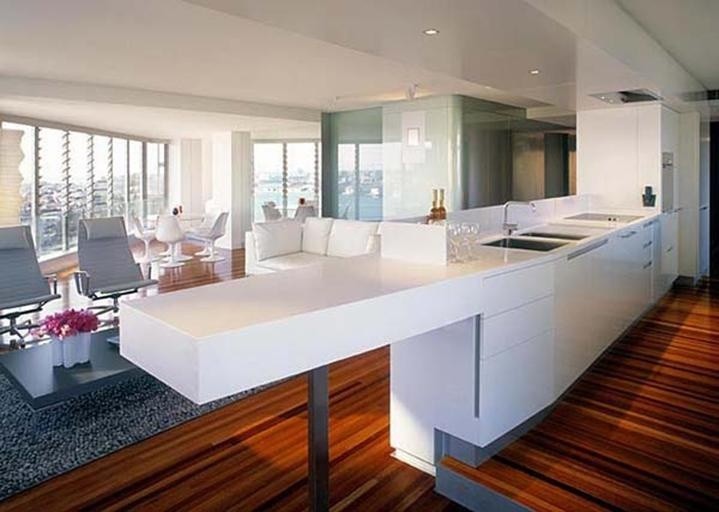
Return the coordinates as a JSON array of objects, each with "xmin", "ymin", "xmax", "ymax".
[
  {"xmin": 438, "ymin": 189, "xmax": 447, "ymax": 220},
  {"xmin": 426, "ymin": 189, "xmax": 440, "ymax": 227}
]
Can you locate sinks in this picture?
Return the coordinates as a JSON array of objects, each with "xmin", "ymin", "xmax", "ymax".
[
  {"xmin": 519, "ymin": 232, "xmax": 588, "ymax": 240},
  {"xmin": 481, "ymin": 237, "xmax": 570, "ymax": 252}
]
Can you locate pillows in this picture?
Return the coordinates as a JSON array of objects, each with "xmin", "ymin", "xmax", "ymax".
[{"xmin": 248, "ymin": 218, "xmax": 302, "ymax": 261}]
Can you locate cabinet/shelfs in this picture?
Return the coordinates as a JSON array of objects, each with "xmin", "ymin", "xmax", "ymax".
[
  {"xmin": 620, "ymin": 219, "xmax": 670, "ymax": 328},
  {"xmin": 384, "ymin": 260, "xmax": 555, "ymax": 480}
]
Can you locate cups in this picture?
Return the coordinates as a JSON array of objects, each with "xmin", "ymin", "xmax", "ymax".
[{"xmin": 445, "ymin": 222, "xmax": 484, "ymax": 264}]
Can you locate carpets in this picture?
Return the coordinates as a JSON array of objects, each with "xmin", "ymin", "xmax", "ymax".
[{"xmin": 0, "ymin": 352, "xmax": 302, "ymax": 501}]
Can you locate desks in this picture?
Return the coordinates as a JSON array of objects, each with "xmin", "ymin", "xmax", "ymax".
[
  {"xmin": 1, "ymin": 326, "xmax": 142, "ymax": 409},
  {"xmin": 117, "ymin": 271, "xmax": 476, "ymax": 512}
]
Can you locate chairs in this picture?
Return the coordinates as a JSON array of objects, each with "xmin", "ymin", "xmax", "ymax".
[
  {"xmin": 125, "ymin": 197, "xmax": 231, "ymax": 269},
  {"xmin": 0, "ymin": 223, "xmax": 64, "ymax": 352},
  {"xmin": 71, "ymin": 216, "xmax": 160, "ymax": 329},
  {"xmin": 260, "ymin": 200, "xmax": 320, "ymax": 221}
]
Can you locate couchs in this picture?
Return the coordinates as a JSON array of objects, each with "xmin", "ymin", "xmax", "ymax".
[{"xmin": 243, "ymin": 216, "xmax": 378, "ymax": 277}]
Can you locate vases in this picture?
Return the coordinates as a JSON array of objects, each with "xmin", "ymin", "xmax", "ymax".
[{"xmin": 52, "ymin": 332, "xmax": 97, "ymax": 370}]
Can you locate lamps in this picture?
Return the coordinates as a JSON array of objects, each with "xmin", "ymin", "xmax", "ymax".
[{"xmin": 403, "ymin": 80, "xmax": 421, "ymax": 101}]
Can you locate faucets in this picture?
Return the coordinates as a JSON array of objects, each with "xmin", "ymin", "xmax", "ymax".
[{"xmin": 502, "ymin": 200, "xmax": 536, "ymax": 233}]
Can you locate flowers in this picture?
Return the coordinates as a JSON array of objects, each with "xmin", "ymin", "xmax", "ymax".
[{"xmin": 30, "ymin": 305, "xmax": 100, "ymax": 339}]
